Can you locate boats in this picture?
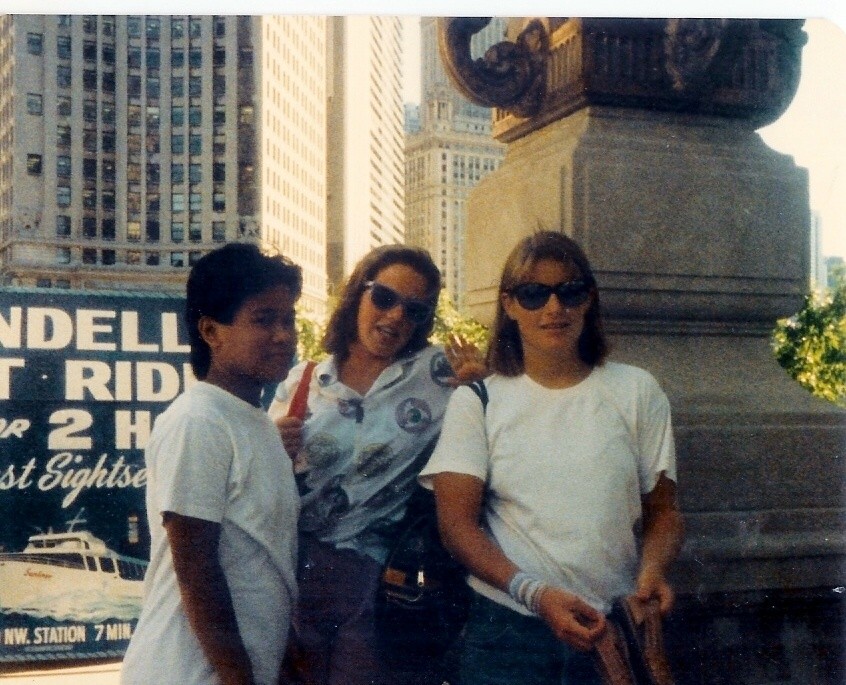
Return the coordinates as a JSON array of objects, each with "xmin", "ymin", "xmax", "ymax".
[{"xmin": 0, "ymin": 509, "xmax": 150, "ymax": 582}]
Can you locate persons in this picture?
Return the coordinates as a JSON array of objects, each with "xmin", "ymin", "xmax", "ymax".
[
  {"xmin": 416, "ymin": 229, "xmax": 688, "ymax": 685},
  {"xmin": 119, "ymin": 246, "xmax": 301, "ymax": 685},
  {"xmin": 266, "ymin": 246, "xmax": 489, "ymax": 685}
]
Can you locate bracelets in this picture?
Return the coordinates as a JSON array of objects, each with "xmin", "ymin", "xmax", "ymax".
[{"xmin": 508, "ymin": 572, "xmax": 548, "ymax": 616}]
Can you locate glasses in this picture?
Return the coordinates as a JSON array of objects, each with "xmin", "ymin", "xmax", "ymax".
[
  {"xmin": 504, "ymin": 280, "xmax": 594, "ymax": 311},
  {"xmin": 364, "ymin": 280, "xmax": 435, "ymax": 327}
]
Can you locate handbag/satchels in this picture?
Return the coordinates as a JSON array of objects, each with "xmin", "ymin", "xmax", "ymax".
[{"xmin": 374, "ymin": 508, "xmax": 472, "ymax": 672}]
